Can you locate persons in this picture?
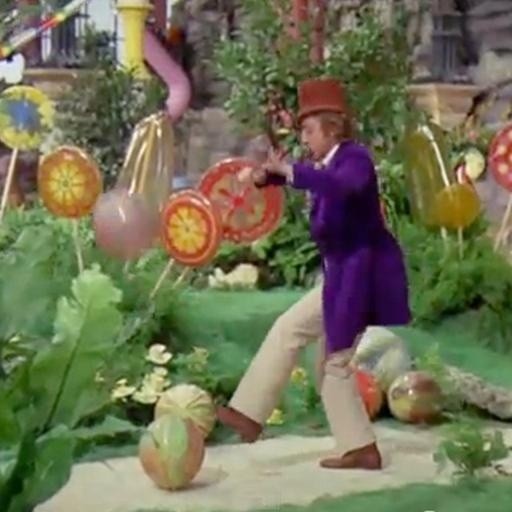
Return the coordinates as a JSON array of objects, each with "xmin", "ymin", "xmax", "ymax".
[{"xmin": 216, "ymin": 77, "xmax": 412, "ymax": 470}]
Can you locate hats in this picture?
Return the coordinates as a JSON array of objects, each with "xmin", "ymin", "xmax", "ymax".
[{"xmin": 293, "ymin": 76, "xmax": 348, "ymax": 129}]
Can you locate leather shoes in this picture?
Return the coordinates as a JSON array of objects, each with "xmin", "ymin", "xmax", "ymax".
[
  {"xmin": 212, "ymin": 403, "xmax": 263, "ymax": 443},
  {"xmin": 319, "ymin": 442, "xmax": 384, "ymax": 471}
]
